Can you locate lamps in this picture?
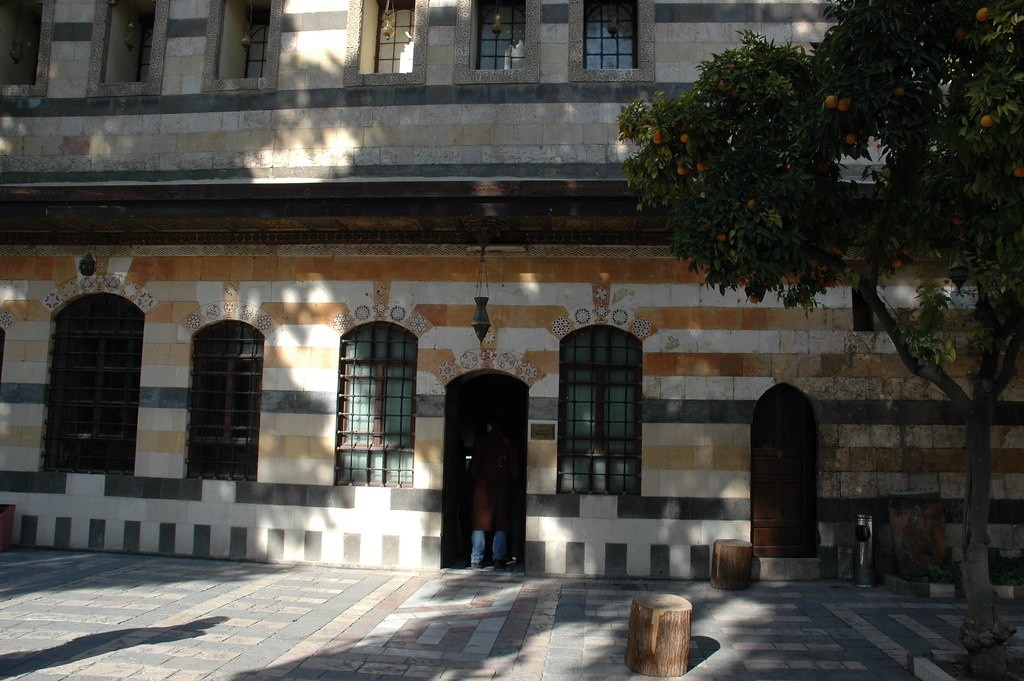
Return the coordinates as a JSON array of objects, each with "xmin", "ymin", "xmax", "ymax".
[
  {"xmin": 380, "ymin": 17, "xmax": 396, "ymax": 41},
  {"xmin": 241, "ymin": 30, "xmax": 254, "ymax": 51},
  {"xmin": 124, "ymin": 22, "xmax": 137, "ymax": 52},
  {"xmin": 607, "ymin": 15, "xmax": 620, "ymax": 39},
  {"xmin": 8, "ymin": 34, "xmax": 26, "ymax": 66},
  {"xmin": 491, "ymin": 14, "xmax": 505, "ymax": 37},
  {"xmin": 78, "ymin": 223, "xmax": 97, "ymax": 278},
  {"xmin": 944, "ymin": 250, "xmax": 972, "ymax": 297},
  {"xmin": 471, "ymin": 253, "xmax": 492, "ymax": 347}
]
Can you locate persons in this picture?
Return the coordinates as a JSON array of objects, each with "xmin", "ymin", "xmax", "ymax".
[{"xmin": 470, "ymin": 413, "xmax": 528, "ymax": 572}]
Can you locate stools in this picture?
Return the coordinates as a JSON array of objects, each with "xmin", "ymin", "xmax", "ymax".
[
  {"xmin": 710, "ymin": 539, "xmax": 752, "ymax": 590},
  {"xmin": 625, "ymin": 592, "xmax": 694, "ymax": 680}
]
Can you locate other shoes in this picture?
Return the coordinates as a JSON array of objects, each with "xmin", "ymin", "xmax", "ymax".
[
  {"xmin": 506, "ymin": 559, "xmax": 523, "ymax": 566},
  {"xmin": 471, "ymin": 558, "xmax": 493, "ymax": 568},
  {"xmin": 494, "ymin": 559, "xmax": 506, "ymax": 569}
]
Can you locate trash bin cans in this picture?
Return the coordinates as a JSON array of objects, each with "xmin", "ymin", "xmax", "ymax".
[{"xmin": 852, "ymin": 512, "xmax": 877, "ymax": 589}]
